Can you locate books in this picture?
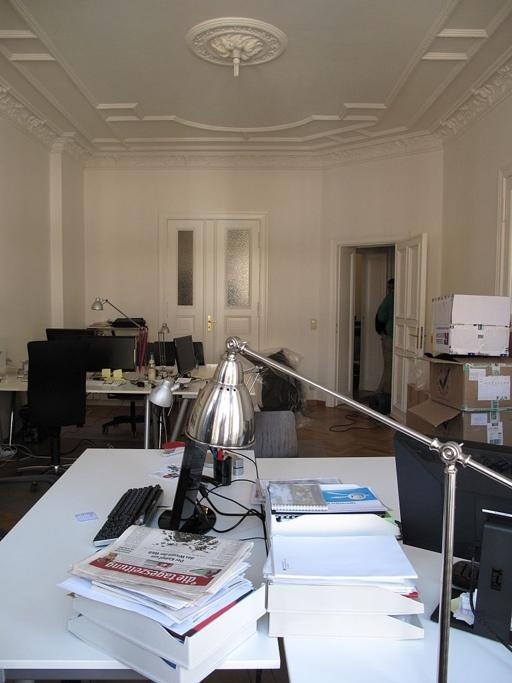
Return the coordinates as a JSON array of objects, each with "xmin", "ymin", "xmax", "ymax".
[
  {"xmin": 260, "ymin": 481, "xmax": 424, "ymax": 640},
  {"xmin": 62, "ymin": 527, "xmax": 267, "ymax": 683}
]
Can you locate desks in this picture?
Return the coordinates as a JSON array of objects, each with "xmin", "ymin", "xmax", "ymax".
[{"xmin": 1, "ymin": 362, "xmax": 254, "ymax": 449}]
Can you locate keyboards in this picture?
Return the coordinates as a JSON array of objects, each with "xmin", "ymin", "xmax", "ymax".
[{"xmin": 93, "ymin": 483, "xmax": 164, "ymax": 546}]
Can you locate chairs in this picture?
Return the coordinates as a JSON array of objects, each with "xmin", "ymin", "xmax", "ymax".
[
  {"xmin": 16, "ymin": 341, "xmax": 89, "ymax": 485},
  {"xmin": 101, "ymin": 390, "xmax": 152, "ymax": 438}
]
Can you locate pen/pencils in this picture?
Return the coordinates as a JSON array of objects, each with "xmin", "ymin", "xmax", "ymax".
[{"xmin": 210, "ymin": 447, "xmax": 229, "ymax": 460}]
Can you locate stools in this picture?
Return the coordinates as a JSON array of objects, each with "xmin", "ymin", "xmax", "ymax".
[{"xmin": 254, "ymin": 410, "xmax": 298, "ymax": 458}]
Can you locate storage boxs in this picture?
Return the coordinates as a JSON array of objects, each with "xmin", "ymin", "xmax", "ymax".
[{"xmin": 407, "ymin": 293, "xmax": 512, "ymax": 447}]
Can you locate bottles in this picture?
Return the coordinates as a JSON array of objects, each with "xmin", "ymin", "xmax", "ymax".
[{"xmin": 148, "ymin": 359, "xmax": 156, "ymax": 383}]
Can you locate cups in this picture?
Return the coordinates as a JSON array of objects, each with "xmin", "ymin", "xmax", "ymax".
[{"xmin": 213, "ymin": 455, "xmax": 232, "ymax": 486}]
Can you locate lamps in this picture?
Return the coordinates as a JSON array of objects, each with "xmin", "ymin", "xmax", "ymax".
[
  {"xmin": 158, "ymin": 322, "xmax": 170, "ymax": 366},
  {"xmin": 184, "ymin": 338, "xmax": 512, "ymax": 683},
  {"xmin": 91, "ymin": 297, "xmax": 148, "ymax": 376}
]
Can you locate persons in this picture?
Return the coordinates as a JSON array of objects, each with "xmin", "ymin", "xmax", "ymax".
[{"xmin": 374, "ymin": 277, "xmax": 393, "ymax": 394}]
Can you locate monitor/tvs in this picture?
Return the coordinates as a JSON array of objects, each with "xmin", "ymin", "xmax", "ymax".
[
  {"xmin": 156, "ymin": 432, "xmax": 216, "ymax": 535},
  {"xmin": 393, "ymin": 430, "xmax": 511, "ymax": 647},
  {"xmin": 44, "ymin": 326, "xmax": 206, "ymax": 388}
]
